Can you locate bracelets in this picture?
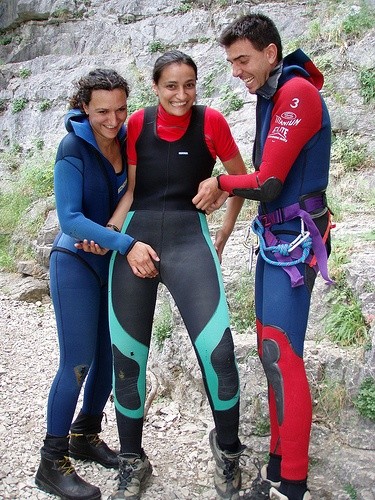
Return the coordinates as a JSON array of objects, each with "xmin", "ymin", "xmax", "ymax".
[{"xmin": 106, "ymin": 223, "xmax": 119, "ymax": 232}]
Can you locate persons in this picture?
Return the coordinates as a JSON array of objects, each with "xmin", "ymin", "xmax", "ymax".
[
  {"xmin": 74, "ymin": 49, "xmax": 251, "ymax": 500},
  {"xmin": 192, "ymin": 14, "xmax": 336, "ymax": 500},
  {"xmin": 34, "ymin": 68, "xmax": 160, "ymax": 500}
]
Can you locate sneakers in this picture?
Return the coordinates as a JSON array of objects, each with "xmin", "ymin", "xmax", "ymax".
[
  {"xmin": 111, "ymin": 449, "xmax": 152, "ymax": 500},
  {"xmin": 209, "ymin": 428, "xmax": 249, "ymax": 498}
]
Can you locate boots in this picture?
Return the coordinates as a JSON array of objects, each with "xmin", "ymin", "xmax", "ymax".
[
  {"xmin": 68, "ymin": 432, "xmax": 120, "ymax": 468},
  {"xmin": 34, "ymin": 435, "xmax": 100, "ymax": 500}
]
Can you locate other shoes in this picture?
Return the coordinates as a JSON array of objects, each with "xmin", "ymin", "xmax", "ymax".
[{"xmin": 254, "ymin": 464, "xmax": 281, "ymax": 496}]
